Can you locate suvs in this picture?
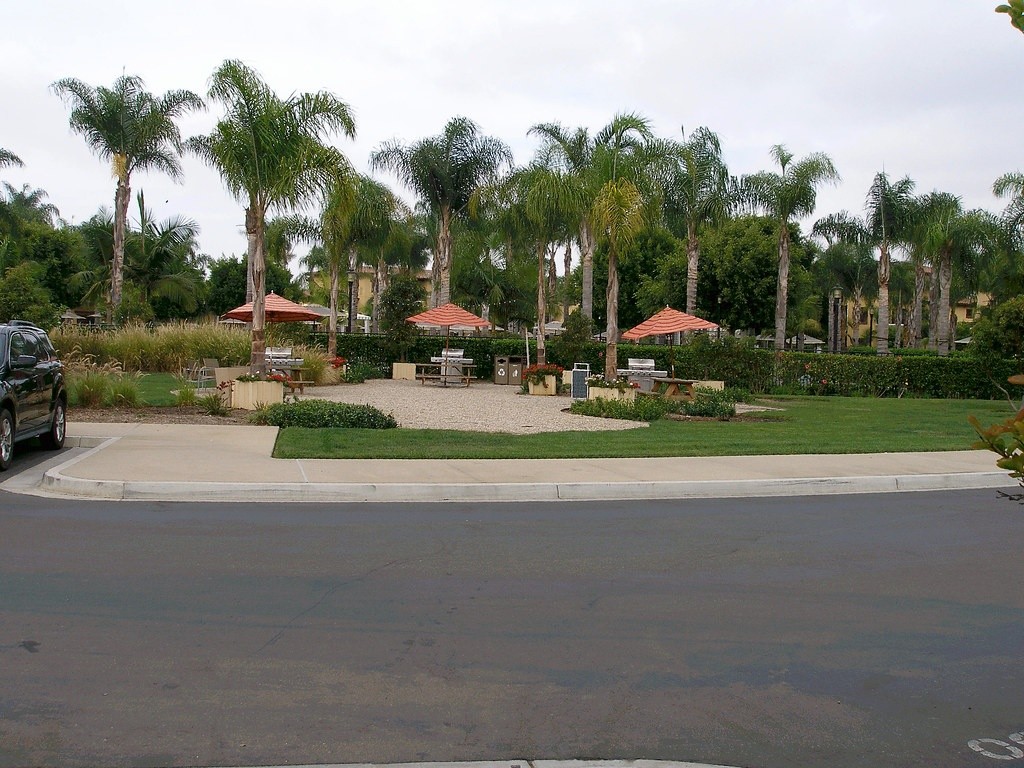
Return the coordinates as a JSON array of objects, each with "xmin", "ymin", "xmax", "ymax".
[{"xmin": 0, "ymin": 318, "xmax": 68, "ymax": 472}]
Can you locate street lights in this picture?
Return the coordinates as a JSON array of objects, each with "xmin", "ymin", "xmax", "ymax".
[
  {"xmin": 868, "ymin": 305, "xmax": 877, "ymax": 345},
  {"xmin": 832, "ymin": 283, "xmax": 843, "ymax": 355},
  {"xmin": 346, "ymin": 266, "xmax": 360, "ymax": 333}
]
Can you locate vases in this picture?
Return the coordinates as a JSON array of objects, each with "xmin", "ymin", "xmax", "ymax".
[
  {"xmin": 589, "ymin": 387, "xmax": 635, "ymax": 404},
  {"xmin": 528, "ymin": 375, "xmax": 557, "ymax": 395}
]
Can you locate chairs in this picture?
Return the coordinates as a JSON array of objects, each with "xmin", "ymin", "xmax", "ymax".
[{"xmin": 186, "ymin": 358, "xmax": 220, "ymax": 396}]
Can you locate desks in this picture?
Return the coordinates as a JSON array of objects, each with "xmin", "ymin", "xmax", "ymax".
[
  {"xmin": 416, "ymin": 363, "xmax": 477, "ymax": 387},
  {"xmin": 652, "ymin": 378, "xmax": 699, "ymax": 400},
  {"xmin": 266, "ymin": 366, "xmax": 309, "ymax": 394}
]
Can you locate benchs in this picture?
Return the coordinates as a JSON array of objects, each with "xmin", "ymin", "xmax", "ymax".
[
  {"xmin": 287, "ymin": 381, "xmax": 315, "ymax": 384},
  {"xmin": 684, "ymin": 391, "xmax": 711, "ymax": 396},
  {"xmin": 416, "ymin": 374, "xmax": 477, "ymax": 379},
  {"xmin": 647, "ymin": 392, "xmax": 669, "ymax": 396}
]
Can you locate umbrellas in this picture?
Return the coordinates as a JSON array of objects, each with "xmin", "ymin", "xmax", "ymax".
[
  {"xmin": 621, "ymin": 304, "xmax": 720, "ymax": 379},
  {"xmin": 405, "ymin": 303, "xmax": 493, "ymax": 385},
  {"xmin": 221, "ymin": 290, "xmax": 321, "ymax": 373}
]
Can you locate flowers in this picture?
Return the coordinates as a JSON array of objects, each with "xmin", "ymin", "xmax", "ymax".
[
  {"xmin": 517, "ymin": 363, "xmax": 565, "ymax": 394},
  {"xmin": 584, "ymin": 374, "xmax": 639, "ymax": 394}
]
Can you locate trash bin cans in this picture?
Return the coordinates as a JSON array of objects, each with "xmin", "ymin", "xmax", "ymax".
[
  {"xmin": 495, "ymin": 355, "xmax": 523, "ymax": 385},
  {"xmin": 571, "ymin": 362, "xmax": 592, "ymax": 399}
]
